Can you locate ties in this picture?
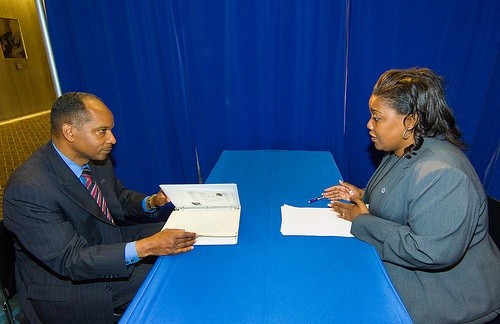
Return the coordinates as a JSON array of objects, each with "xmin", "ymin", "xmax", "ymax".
[{"xmin": 82, "ymin": 167, "xmax": 116, "ymax": 226}]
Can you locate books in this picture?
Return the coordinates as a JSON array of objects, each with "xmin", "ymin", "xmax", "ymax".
[{"xmin": 158, "ymin": 183, "xmax": 241, "ymax": 246}]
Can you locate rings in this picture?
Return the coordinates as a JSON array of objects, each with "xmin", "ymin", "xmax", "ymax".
[{"xmin": 342, "ymin": 212, "xmax": 346, "ymax": 217}]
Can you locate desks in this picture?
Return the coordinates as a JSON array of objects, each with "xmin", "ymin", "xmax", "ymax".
[{"xmin": 117, "ymin": 149, "xmax": 413, "ymax": 324}]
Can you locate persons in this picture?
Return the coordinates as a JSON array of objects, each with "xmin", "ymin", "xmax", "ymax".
[
  {"xmin": 320, "ymin": 68, "xmax": 500, "ymax": 324},
  {"xmin": 3, "ymin": 91, "xmax": 198, "ymax": 324}
]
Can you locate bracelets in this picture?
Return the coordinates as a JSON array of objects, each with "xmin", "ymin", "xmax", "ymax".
[{"xmin": 147, "ymin": 193, "xmax": 158, "ymax": 209}]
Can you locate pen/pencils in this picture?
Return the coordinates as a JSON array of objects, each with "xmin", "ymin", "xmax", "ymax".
[{"xmin": 308, "ymin": 196, "xmax": 325, "ymax": 204}]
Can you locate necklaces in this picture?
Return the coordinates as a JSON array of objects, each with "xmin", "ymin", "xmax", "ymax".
[{"xmin": 364, "ymin": 151, "xmax": 405, "ymax": 205}]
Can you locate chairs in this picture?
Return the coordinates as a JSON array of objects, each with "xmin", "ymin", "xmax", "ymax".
[{"xmin": 0, "ymin": 221, "xmax": 123, "ymax": 324}]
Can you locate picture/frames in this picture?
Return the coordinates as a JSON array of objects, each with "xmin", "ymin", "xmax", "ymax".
[{"xmin": 0, "ymin": 17, "xmax": 28, "ymax": 61}]
